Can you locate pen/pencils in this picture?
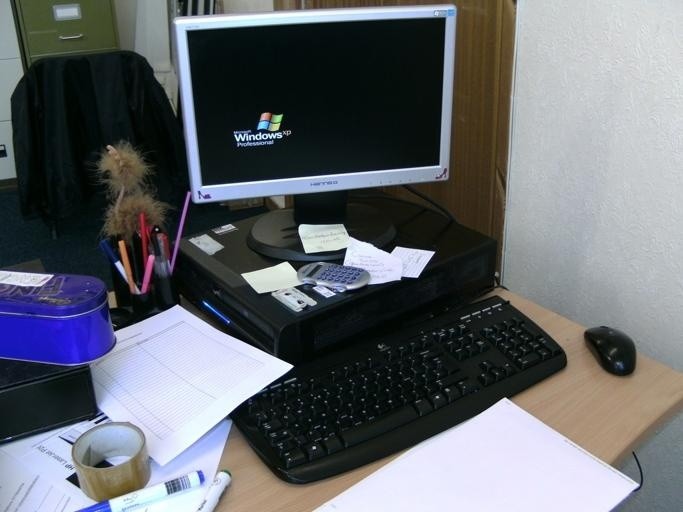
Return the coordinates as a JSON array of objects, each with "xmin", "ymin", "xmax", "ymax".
[{"xmin": 102, "ymin": 190, "xmax": 191, "ymax": 295}]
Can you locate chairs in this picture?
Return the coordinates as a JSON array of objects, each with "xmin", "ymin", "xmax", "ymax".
[{"xmin": 9, "ymin": 51, "xmax": 200, "ymax": 239}]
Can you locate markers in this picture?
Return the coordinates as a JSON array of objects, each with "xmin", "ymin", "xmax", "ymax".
[{"xmin": 73, "ymin": 471, "xmax": 231, "ymax": 512}]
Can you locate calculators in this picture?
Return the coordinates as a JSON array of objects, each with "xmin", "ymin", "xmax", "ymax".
[{"xmin": 297, "ymin": 262, "xmax": 371, "ymax": 291}]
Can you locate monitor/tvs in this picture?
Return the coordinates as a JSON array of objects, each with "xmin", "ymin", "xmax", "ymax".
[{"xmin": 171, "ymin": 4, "xmax": 458, "ymax": 262}]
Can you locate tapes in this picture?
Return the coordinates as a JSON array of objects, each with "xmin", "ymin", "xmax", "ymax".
[{"xmin": 71, "ymin": 421, "xmax": 151, "ymax": 503}]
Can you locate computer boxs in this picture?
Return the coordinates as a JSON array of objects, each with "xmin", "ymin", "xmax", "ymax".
[{"xmin": 170, "ymin": 190, "xmax": 498, "ymax": 366}]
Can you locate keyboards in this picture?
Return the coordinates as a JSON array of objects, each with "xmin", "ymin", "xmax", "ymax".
[{"xmin": 229, "ymin": 295, "xmax": 568, "ymax": 485}]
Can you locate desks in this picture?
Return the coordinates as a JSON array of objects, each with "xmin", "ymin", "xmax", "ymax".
[{"xmin": 0, "ymin": 289, "xmax": 683, "ymax": 512}]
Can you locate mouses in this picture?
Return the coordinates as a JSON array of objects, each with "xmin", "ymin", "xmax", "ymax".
[{"xmin": 584, "ymin": 325, "xmax": 637, "ymax": 377}]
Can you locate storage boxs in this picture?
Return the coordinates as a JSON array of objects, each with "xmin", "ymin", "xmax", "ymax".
[{"xmin": 0, "ymin": 258, "xmax": 117, "ymax": 441}]
